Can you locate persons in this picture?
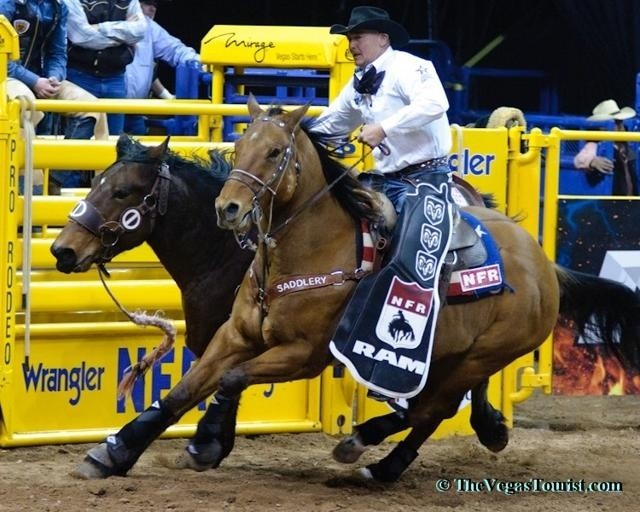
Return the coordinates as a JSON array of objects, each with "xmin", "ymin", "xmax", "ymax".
[
  {"xmin": 0, "ymin": 0, "xmax": 210, "ymax": 197},
  {"xmin": 301, "ymin": 4, "xmax": 460, "ymax": 401},
  {"xmin": 571, "ymin": 99, "xmax": 640, "ymax": 205}
]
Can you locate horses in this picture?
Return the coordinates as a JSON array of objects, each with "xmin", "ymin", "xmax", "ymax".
[
  {"xmin": 49, "ymin": 132, "xmax": 510, "ymax": 475},
  {"xmin": 68, "ymin": 90, "xmax": 639, "ymax": 491}
]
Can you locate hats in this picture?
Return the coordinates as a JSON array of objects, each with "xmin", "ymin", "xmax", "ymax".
[
  {"xmin": 330, "ymin": 6, "xmax": 409, "ymax": 44},
  {"xmin": 586, "ymin": 99, "xmax": 635, "ymax": 123}
]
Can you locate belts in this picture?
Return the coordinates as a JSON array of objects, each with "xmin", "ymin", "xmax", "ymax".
[{"xmin": 384, "ymin": 156, "xmax": 448, "ymax": 178}]
